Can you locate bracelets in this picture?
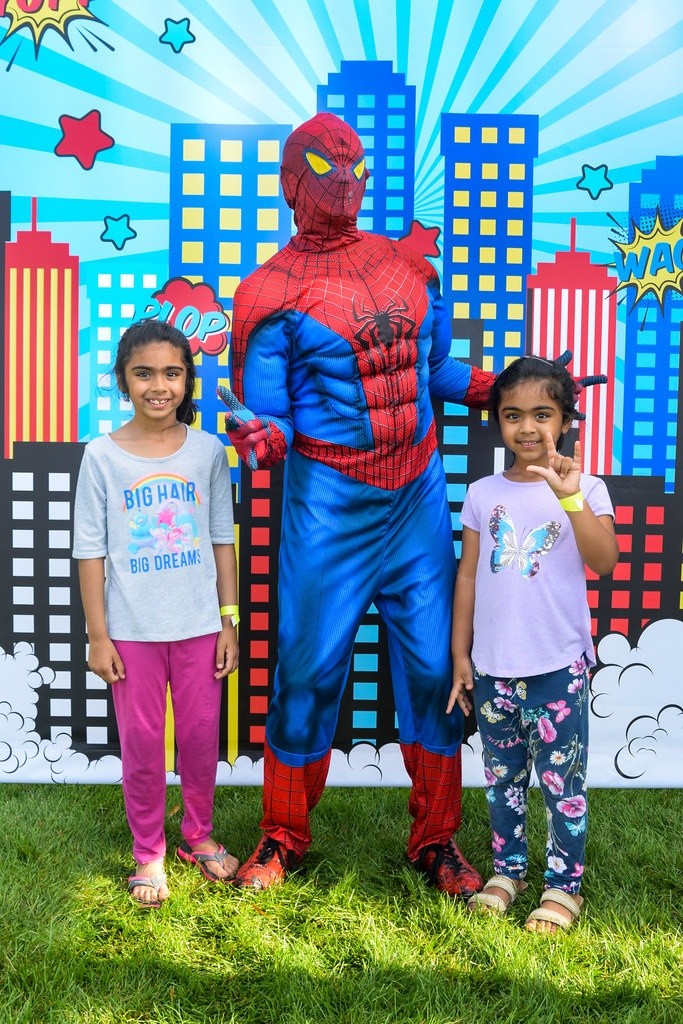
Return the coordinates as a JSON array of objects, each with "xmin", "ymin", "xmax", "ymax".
[
  {"xmin": 220, "ymin": 605, "xmax": 240, "ymax": 628},
  {"xmin": 559, "ymin": 490, "xmax": 585, "ymax": 512}
]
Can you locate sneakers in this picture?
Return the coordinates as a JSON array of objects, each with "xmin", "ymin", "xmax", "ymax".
[
  {"xmin": 234, "ymin": 834, "xmax": 303, "ymax": 890},
  {"xmin": 422, "ymin": 839, "xmax": 482, "ymax": 897}
]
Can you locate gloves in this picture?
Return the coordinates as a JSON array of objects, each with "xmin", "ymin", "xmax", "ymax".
[
  {"xmin": 554, "ymin": 350, "xmax": 609, "ymax": 422},
  {"xmin": 218, "ymin": 386, "xmax": 270, "ymax": 470}
]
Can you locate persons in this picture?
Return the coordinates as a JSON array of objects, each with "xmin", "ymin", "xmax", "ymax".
[
  {"xmin": 71, "ymin": 319, "xmax": 240, "ymax": 905},
  {"xmin": 447, "ymin": 354, "xmax": 620, "ymax": 932},
  {"xmin": 215, "ymin": 112, "xmax": 608, "ymax": 900}
]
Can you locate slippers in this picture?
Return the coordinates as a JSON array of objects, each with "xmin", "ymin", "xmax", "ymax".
[
  {"xmin": 526, "ymin": 888, "xmax": 586, "ymax": 935},
  {"xmin": 177, "ymin": 841, "xmax": 236, "ymax": 882},
  {"xmin": 467, "ymin": 874, "xmax": 528, "ymax": 916},
  {"xmin": 129, "ymin": 871, "xmax": 168, "ymax": 908}
]
